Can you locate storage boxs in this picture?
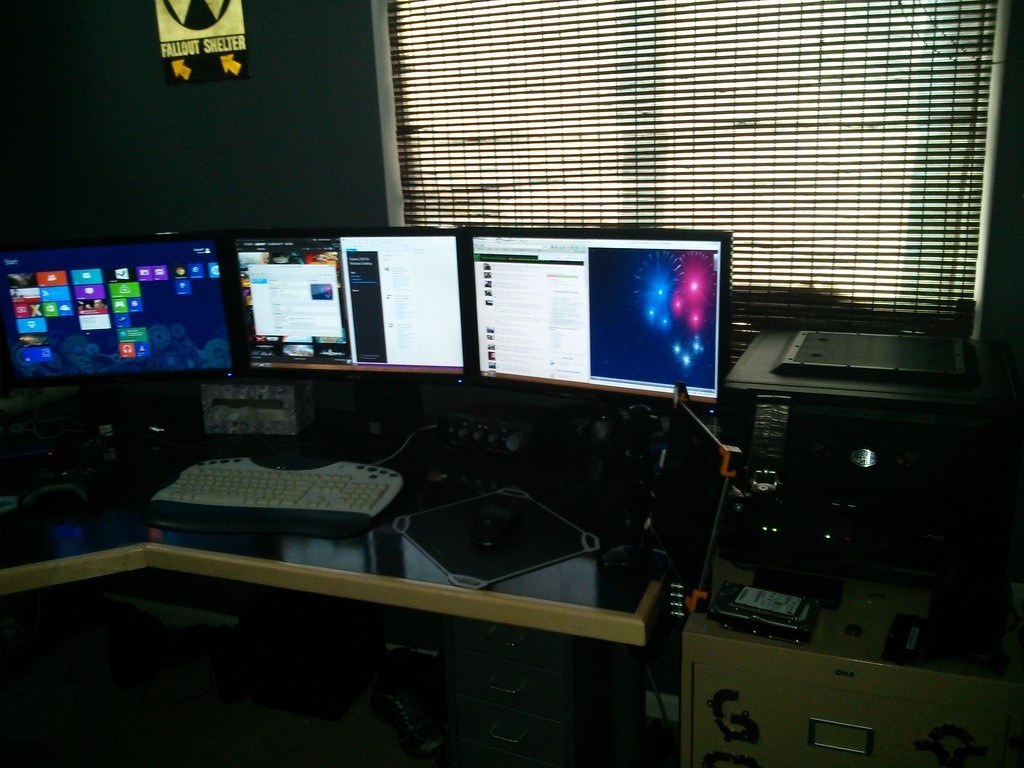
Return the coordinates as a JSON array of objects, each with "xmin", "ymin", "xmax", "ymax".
[{"xmin": 201, "ymin": 377, "xmax": 317, "ymax": 437}]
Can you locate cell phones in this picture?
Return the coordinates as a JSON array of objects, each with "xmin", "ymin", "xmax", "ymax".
[{"xmin": 728, "ymin": 586, "xmax": 808, "ymax": 621}]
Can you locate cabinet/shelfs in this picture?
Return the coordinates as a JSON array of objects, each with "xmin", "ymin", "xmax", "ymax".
[
  {"xmin": 678, "ymin": 552, "xmax": 1024, "ymax": 768},
  {"xmin": 442, "ymin": 613, "xmax": 618, "ymax": 768}
]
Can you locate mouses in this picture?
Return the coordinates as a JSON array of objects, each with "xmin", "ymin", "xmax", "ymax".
[{"xmin": 475, "ymin": 513, "xmax": 506, "ymax": 547}]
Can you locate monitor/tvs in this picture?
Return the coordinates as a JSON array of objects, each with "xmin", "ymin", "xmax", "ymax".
[{"xmin": 0, "ymin": 225, "xmax": 731, "ymax": 448}]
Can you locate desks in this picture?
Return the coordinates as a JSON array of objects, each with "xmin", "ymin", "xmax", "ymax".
[{"xmin": 0, "ymin": 399, "xmax": 711, "ymax": 768}]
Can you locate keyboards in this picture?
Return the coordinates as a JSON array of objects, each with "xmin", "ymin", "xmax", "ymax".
[{"xmin": 140, "ymin": 457, "xmax": 405, "ymax": 540}]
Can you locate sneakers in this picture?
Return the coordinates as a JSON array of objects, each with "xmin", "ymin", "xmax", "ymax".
[{"xmin": 370, "ymin": 646, "xmax": 450, "ymax": 761}]
